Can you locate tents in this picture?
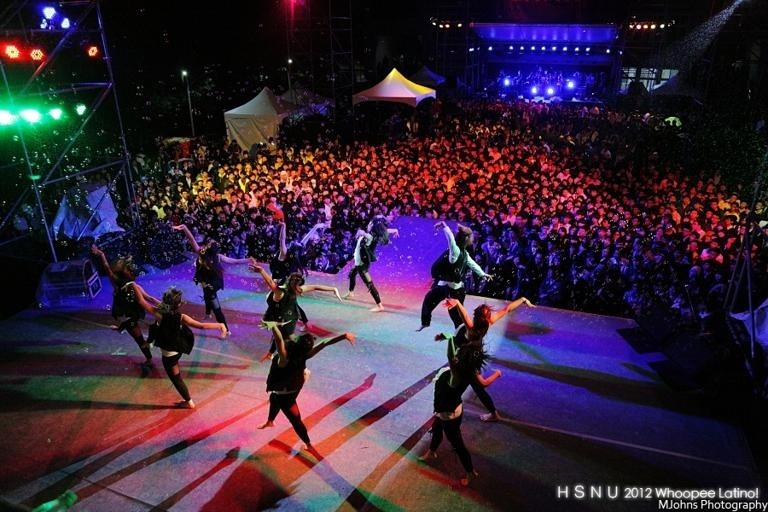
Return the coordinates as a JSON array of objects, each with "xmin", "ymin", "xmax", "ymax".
[
  {"xmin": 280, "ymin": 82, "xmax": 336, "ymax": 117},
  {"xmin": 223, "ymin": 87, "xmax": 307, "ymax": 152}
]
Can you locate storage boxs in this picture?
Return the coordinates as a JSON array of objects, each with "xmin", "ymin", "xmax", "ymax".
[{"xmin": 39, "ymin": 258, "xmax": 102, "ymax": 304}]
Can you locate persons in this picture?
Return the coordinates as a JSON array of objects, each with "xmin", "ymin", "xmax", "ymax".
[
  {"xmin": 120, "ymin": 281, "xmax": 227, "ymax": 410},
  {"xmin": 496, "ymin": 218, "xmax": 768, "ymax": 312},
  {"xmin": 267, "ymin": 137, "xmax": 401, "ymax": 220},
  {"xmin": 509, "ymin": 96, "xmax": 768, "ymax": 218},
  {"xmin": 257, "ymin": 223, "xmax": 269, "ymax": 262},
  {"xmin": 89, "ymin": 244, "xmax": 154, "ymax": 373},
  {"xmin": 256, "ymin": 318, "xmax": 355, "ymax": 455},
  {"xmin": 326, "ymin": 220, "xmax": 341, "ymax": 274},
  {"xmin": 134, "ymin": 139, "xmax": 267, "ymax": 223},
  {"xmin": 342, "ymin": 216, "xmax": 400, "ymax": 314},
  {"xmin": 170, "ymin": 224, "xmax": 257, "ymax": 263},
  {"xmin": 419, "ymin": 332, "xmax": 502, "ymax": 487},
  {"xmin": 269, "ymin": 219, "xmax": 325, "ymax": 303},
  {"xmin": 410, "ymin": 219, "xmax": 496, "ymax": 334},
  {"xmin": 402, "ymin": 96, "xmax": 509, "ymax": 219},
  {"xmin": 441, "ymin": 296, "xmax": 537, "ymax": 424},
  {"xmin": 13, "ymin": 131, "xmax": 133, "ymax": 232},
  {"xmin": 170, "ymin": 224, "xmax": 257, "ymax": 340},
  {"xmin": 247, "ymin": 263, "xmax": 342, "ymax": 367},
  {"xmin": 464, "ymin": 219, "xmax": 496, "ymax": 295}
]
